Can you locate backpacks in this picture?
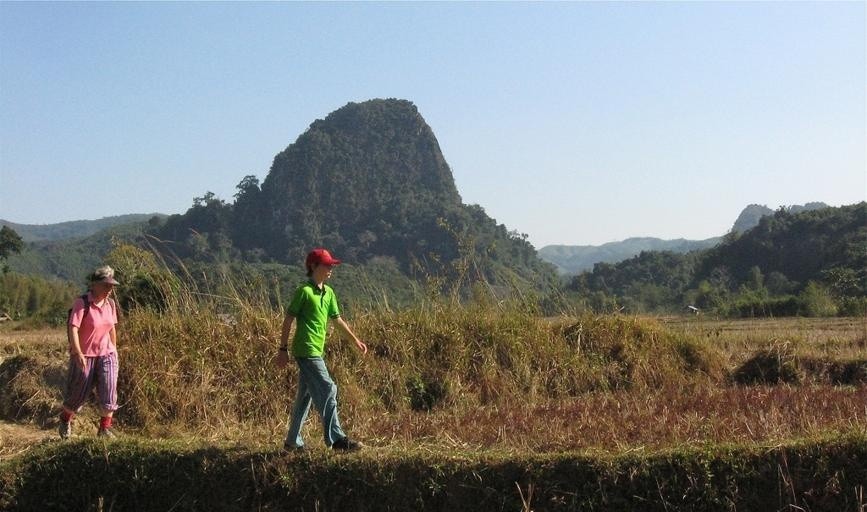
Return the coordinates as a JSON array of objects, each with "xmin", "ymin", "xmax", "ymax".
[{"xmin": 67, "ymin": 294, "xmax": 89, "ymax": 344}]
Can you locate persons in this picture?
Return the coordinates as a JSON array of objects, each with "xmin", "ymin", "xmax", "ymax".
[
  {"xmin": 277, "ymin": 250, "xmax": 369, "ymax": 453},
  {"xmin": 58, "ymin": 267, "xmax": 120, "ymax": 442}
]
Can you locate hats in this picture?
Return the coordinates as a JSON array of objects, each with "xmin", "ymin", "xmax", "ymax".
[
  {"xmin": 306, "ymin": 248, "xmax": 342, "ymax": 266},
  {"xmin": 88, "ymin": 266, "xmax": 120, "ymax": 286}
]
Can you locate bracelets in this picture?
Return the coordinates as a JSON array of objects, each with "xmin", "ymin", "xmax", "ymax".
[
  {"xmin": 75, "ymin": 352, "xmax": 82, "ymax": 356},
  {"xmin": 279, "ymin": 348, "xmax": 288, "ymax": 351}
]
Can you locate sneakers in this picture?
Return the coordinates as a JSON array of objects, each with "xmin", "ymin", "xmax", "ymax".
[
  {"xmin": 283, "ymin": 443, "xmax": 304, "ymax": 453},
  {"xmin": 326, "ymin": 436, "xmax": 364, "ymax": 452},
  {"xmin": 59, "ymin": 414, "xmax": 71, "ymax": 441},
  {"xmin": 97, "ymin": 428, "xmax": 116, "ymax": 439}
]
